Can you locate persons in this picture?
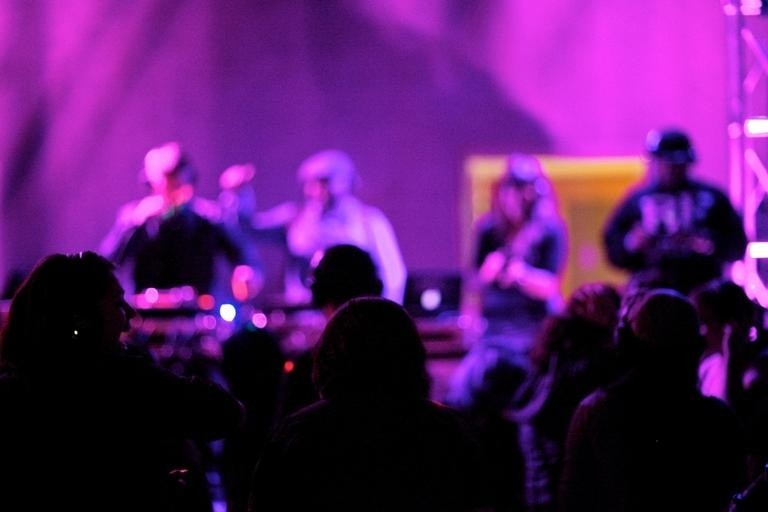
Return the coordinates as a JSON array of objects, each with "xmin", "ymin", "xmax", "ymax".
[
  {"xmin": 218, "ymin": 150, "xmax": 409, "ymax": 305},
  {"xmin": 97, "ymin": 141, "xmax": 264, "ymax": 319},
  {"xmin": 447, "ymin": 156, "xmax": 569, "ymax": 409},
  {"xmin": 602, "ymin": 132, "xmax": 746, "ymax": 300}
]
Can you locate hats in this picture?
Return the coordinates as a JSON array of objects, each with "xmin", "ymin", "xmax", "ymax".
[{"xmin": 649, "ymin": 129, "xmax": 695, "ymax": 165}]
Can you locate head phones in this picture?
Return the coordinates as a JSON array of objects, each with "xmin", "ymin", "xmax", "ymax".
[{"xmin": 611, "ymin": 287, "xmax": 703, "ymax": 362}]
